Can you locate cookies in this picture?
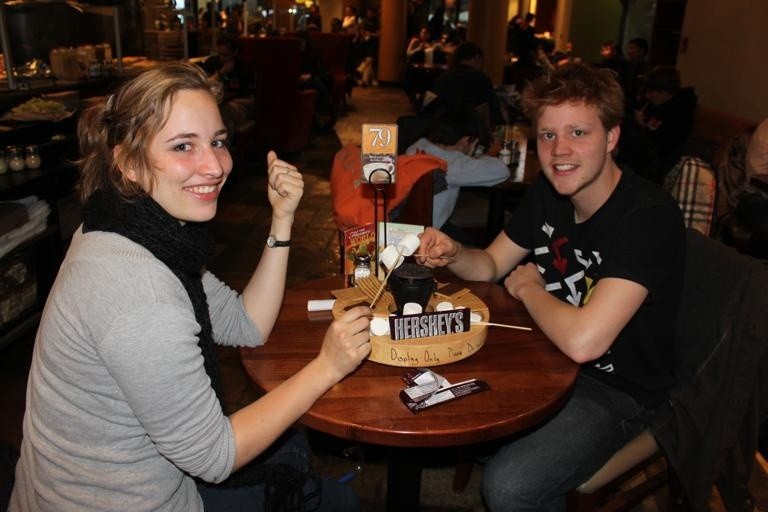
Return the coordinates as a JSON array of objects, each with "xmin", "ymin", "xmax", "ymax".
[
  {"xmin": 434, "ymin": 286, "xmax": 488, "ymax": 313},
  {"xmin": 329, "ymin": 272, "xmax": 400, "ymax": 319}
]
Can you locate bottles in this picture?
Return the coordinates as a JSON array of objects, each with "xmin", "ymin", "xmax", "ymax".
[
  {"xmin": 353, "ymin": 252, "xmax": 371, "ymax": 286},
  {"xmin": 0, "ymin": 144, "xmax": 41, "ymax": 174},
  {"xmin": 87, "ymin": 59, "xmax": 113, "ymax": 80},
  {"xmin": 498, "ymin": 141, "xmax": 519, "ymax": 165}
]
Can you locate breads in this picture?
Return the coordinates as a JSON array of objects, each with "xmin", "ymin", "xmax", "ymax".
[{"xmin": 12, "ymin": 96, "xmax": 66, "ymax": 115}]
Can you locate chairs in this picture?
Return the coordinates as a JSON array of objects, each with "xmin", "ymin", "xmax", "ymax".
[
  {"xmin": 0, "ymin": 35, "xmax": 767, "ymax": 236},
  {"xmin": 572, "ymin": 226, "xmax": 753, "ymax": 511}
]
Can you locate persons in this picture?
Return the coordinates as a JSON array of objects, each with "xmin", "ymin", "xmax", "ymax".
[
  {"xmin": 421, "ymin": 60, "xmax": 685, "ymax": 511},
  {"xmin": 6, "ymin": 60, "xmax": 374, "ymax": 512},
  {"xmin": 191, "ymin": 1, "xmax": 381, "ymax": 145},
  {"xmin": 404, "ymin": 2, "xmax": 767, "ymax": 253}
]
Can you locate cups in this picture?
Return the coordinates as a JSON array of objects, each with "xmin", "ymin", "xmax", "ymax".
[{"xmin": 390, "ymin": 254, "xmax": 434, "ymax": 316}]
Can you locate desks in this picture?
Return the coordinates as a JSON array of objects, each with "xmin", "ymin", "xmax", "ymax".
[{"xmin": 236, "ymin": 267, "xmax": 578, "ymax": 511}]
